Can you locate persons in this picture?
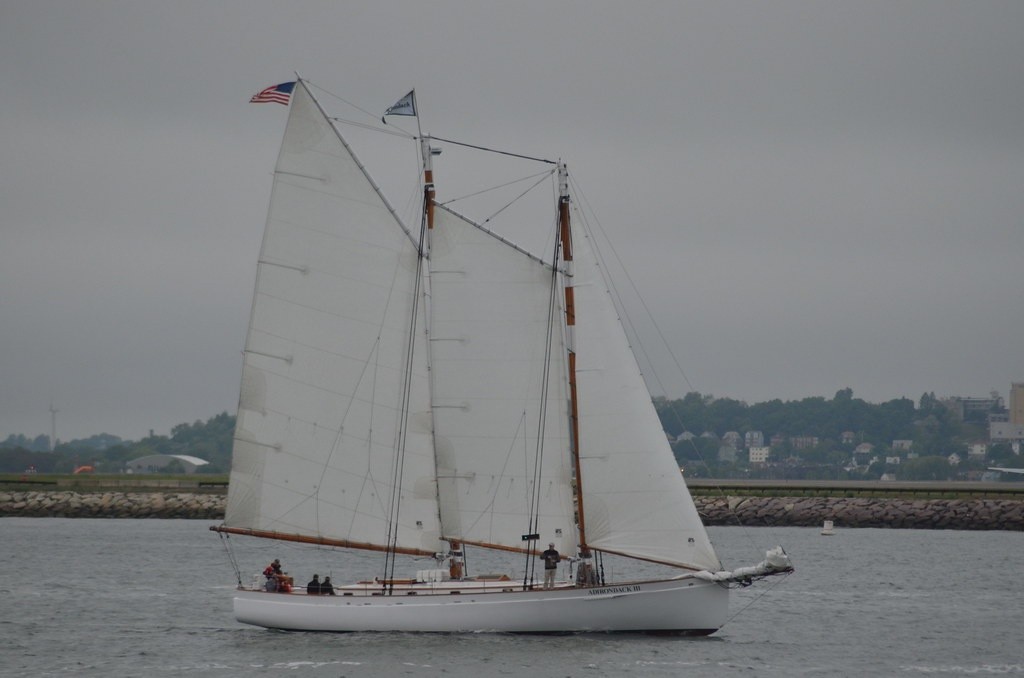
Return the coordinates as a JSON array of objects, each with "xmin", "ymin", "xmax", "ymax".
[
  {"xmin": 540, "ymin": 543, "xmax": 560, "ymax": 590},
  {"xmin": 307, "ymin": 574, "xmax": 320, "ymax": 595},
  {"xmin": 262, "ymin": 559, "xmax": 288, "ymax": 593},
  {"xmin": 320, "ymin": 577, "xmax": 335, "ymax": 594}
]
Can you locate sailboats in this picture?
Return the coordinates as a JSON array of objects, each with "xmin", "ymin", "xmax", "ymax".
[{"xmin": 208, "ymin": 68, "xmax": 796, "ymax": 638}]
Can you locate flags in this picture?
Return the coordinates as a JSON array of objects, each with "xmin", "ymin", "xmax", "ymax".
[
  {"xmin": 380, "ymin": 90, "xmax": 416, "ymax": 124},
  {"xmin": 250, "ymin": 80, "xmax": 295, "ymax": 107}
]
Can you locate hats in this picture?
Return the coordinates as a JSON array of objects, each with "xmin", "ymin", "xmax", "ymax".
[
  {"xmin": 271, "ymin": 560, "xmax": 281, "ymax": 568},
  {"xmin": 548, "ymin": 542, "xmax": 555, "ymax": 547}
]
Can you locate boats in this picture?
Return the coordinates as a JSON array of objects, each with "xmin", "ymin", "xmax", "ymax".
[{"xmin": 820, "ymin": 520, "xmax": 836, "ymax": 535}]
